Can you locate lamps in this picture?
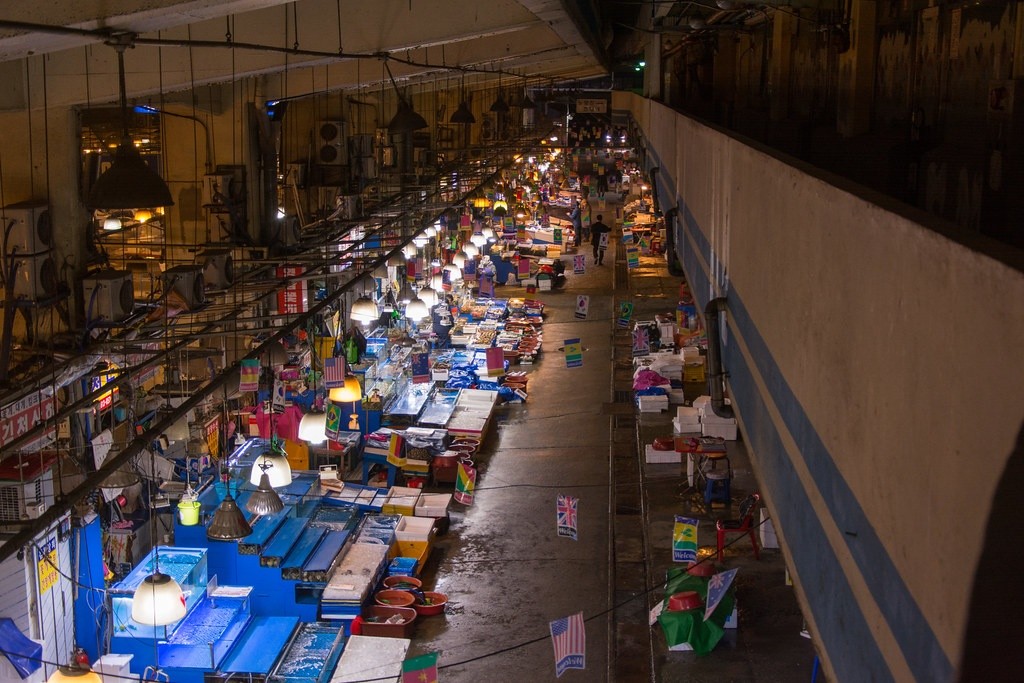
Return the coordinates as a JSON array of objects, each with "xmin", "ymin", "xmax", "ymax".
[
  {"xmin": 130, "ymin": 140, "xmax": 569, "ymax": 626},
  {"xmin": 663, "ymin": 0, "xmax": 850, "ymax": 52},
  {"xmin": 48, "ymin": 650, "xmax": 102, "ymax": 683},
  {"xmin": 619, "ymin": 129, "xmax": 627, "ymax": 142},
  {"xmin": 75, "ymin": 28, "xmax": 176, "ymax": 230},
  {"xmin": 606, "ymin": 127, "xmax": 612, "ymax": 141},
  {"xmin": 387, "ymin": 58, "xmax": 583, "ymax": 135}
]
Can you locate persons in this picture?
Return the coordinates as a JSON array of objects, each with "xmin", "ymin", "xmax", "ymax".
[
  {"xmin": 566, "ymin": 201, "xmax": 581, "ymax": 247},
  {"xmin": 590, "ymin": 214, "xmax": 611, "ymax": 266},
  {"xmin": 596, "ymin": 175, "xmax": 609, "ymax": 197},
  {"xmin": 447, "ymin": 294, "xmax": 459, "ymax": 319},
  {"xmin": 227, "ymin": 415, "xmax": 236, "ymax": 437},
  {"xmin": 581, "ymin": 199, "xmax": 591, "ymax": 242},
  {"xmin": 430, "ymin": 298, "xmax": 454, "ymax": 348}
]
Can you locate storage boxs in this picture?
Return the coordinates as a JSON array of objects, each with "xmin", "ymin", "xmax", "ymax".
[
  {"xmin": 106, "ymin": 160, "xmax": 586, "ymax": 683},
  {"xmin": 616, "ymin": 156, "xmax": 667, "ymax": 247},
  {"xmin": 632, "ymin": 302, "xmax": 738, "ymax": 462}
]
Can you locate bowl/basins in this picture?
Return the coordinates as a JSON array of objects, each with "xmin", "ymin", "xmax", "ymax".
[
  {"xmin": 359, "ymin": 574, "xmax": 448, "ymax": 641},
  {"xmin": 501, "ymin": 376, "xmax": 529, "ymax": 392},
  {"xmin": 668, "ymin": 591, "xmax": 704, "ymax": 611},
  {"xmin": 432, "ymin": 437, "xmax": 481, "ymax": 484},
  {"xmin": 527, "ymin": 316, "xmax": 543, "ymax": 327},
  {"xmin": 652, "ymin": 435, "xmax": 676, "ymax": 451},
  {"xmin": 687, "ymin": 558, "xmax": 717, "ymax": 576},
  {"xmin": 518, "ymin": 337, "xmax": 538, "ymax": 351}
]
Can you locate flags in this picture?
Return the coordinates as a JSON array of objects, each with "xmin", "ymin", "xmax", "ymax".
[
  {"xmin": 672, "ymin": 516, "xmax": 739, "ymax": 619},
  {"xmin": 598, "ymin": 233, "xmax": 608, "ymax": 250},
  {"xmin": 408, "ymin": 257, "xmax": 423, "ymax": 282},
  {"xmin": 411, "ymin": 353, "xmax": 429, "ymax": 384},
  {"xmin": 239, "ymin": 359, "xmax": 259, "ymax": 391},
  {"xmin": 324, "ymin": 356, "xmax": 345, "ymax": 389},
  {"xmin": 549, "ymin": 611, "xmax": 585, "ymax": 677},
  {"xmin": 401, "ymin": 652, "xmax": 439, "ymax": 683},
  {"xmin": 624, "ymin": 231, "xmax": 650, "ymax": 268},
  {"xmin": 442, "ymin": 270, "xmax": 452, "ymax": 292},
  {"xmin": 618, "ymin": 303, "xmax": 649, "ymax": 356},
  {"xmin": 272, "ymin": 377, "xmax": 286, "ymax": 414},
  {"xmin": 324, "ymin": 404, "xmax": 476, "ymax": 505},
  {"xmin": 486, "ymin": 348, "xmax": 505, "ymax": 378},
  {"xmin": 464, "ymin": 260, "xmax": 476, "ymax": 282},
  {"xmin": 574, "ymin": 294, "xmax": 589, "ymax": 320},
  {"xmin": 564, "ymin": 338, "xmax": 583, "ymax": 369},
  {"xmin": 518, "ymin": 259, "xmax": 530, "ymax": 279},
  {"xmin": 462, "ymin": 188, "xmax": 562, "ymax": 245},
  {"xmin": 557, "ymin": 495, "xmax": 580, "ymax": 541},
  {"xmin": 573, "ymin": 255, "xmax": 585, "ymax": 275}
]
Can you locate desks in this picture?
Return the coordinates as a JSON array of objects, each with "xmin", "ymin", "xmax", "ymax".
[
  {"xmin": 675, "ymin": 436, "xmax": 728, "ymax": 487},
  {"xmin": 631, "ymin": 228, "xmax": 650, "ymax": 247}
]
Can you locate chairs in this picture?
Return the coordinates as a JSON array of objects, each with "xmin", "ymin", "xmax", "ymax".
[{"xmin": 716, "ymin": 494, "xmax": 763, "ymax": 561}]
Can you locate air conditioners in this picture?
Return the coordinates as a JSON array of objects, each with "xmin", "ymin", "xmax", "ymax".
[
  {"xmin": 482, "ymin": 128, "xmax": 497, "ymax": 140},
  {"xmin": 0, "ymin": 469, "xmax": 55, "ymax": 520},
  {"xmin": 420, "ymin": 150, "xmax": 437, "ymax": 162},
  {"xmin": 497, "ymin": 122, "xmax": 521, "ymax": 141},
  {"xmin": 0, "ymin": 120, "xmax": 378, "ymax": 324},
  {"xmin": 482, "ymin": 116, "xmax": 496, "ymax": 128},
  {"xmin": 468, "ymin": 144, "xmax": 487, "ymax": 158}
]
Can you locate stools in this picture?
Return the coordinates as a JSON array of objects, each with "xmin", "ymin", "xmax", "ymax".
[
  {"xmin": 651, "ymin": 240, "xmax": 661, "ymax": 254},
  {"xmin": 694, "ymin": 453, "xmax": 734, "ymax": 511}
]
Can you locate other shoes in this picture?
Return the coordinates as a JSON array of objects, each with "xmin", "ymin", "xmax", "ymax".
[
  {"xmin": 594, "ymin": 259, "xmax": 598, "ymax": 265},
  {"xmin": 583, "ymin": 239, "xmax": 588, "ymax": 242},
  {"xmin": 599, "ymin": 263, "xmax": 603, "ymax": 265}
]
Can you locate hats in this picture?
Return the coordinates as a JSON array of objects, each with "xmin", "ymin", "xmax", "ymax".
[{"xmin": 581, "ymin": 199, "xmax": 587, "ymax": 203}]
